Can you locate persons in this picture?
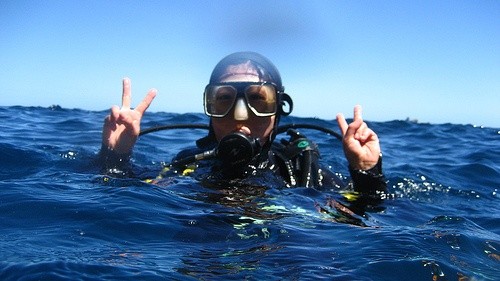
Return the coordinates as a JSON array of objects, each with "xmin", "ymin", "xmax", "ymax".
[{"xmin": 100, "ymin": 51, "xmax": 389, "ymax": 196}]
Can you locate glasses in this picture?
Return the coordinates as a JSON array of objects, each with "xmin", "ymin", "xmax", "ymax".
[{"xmin": 203, "ymin": 81, "xmax": 279, "ymax": 118}]
